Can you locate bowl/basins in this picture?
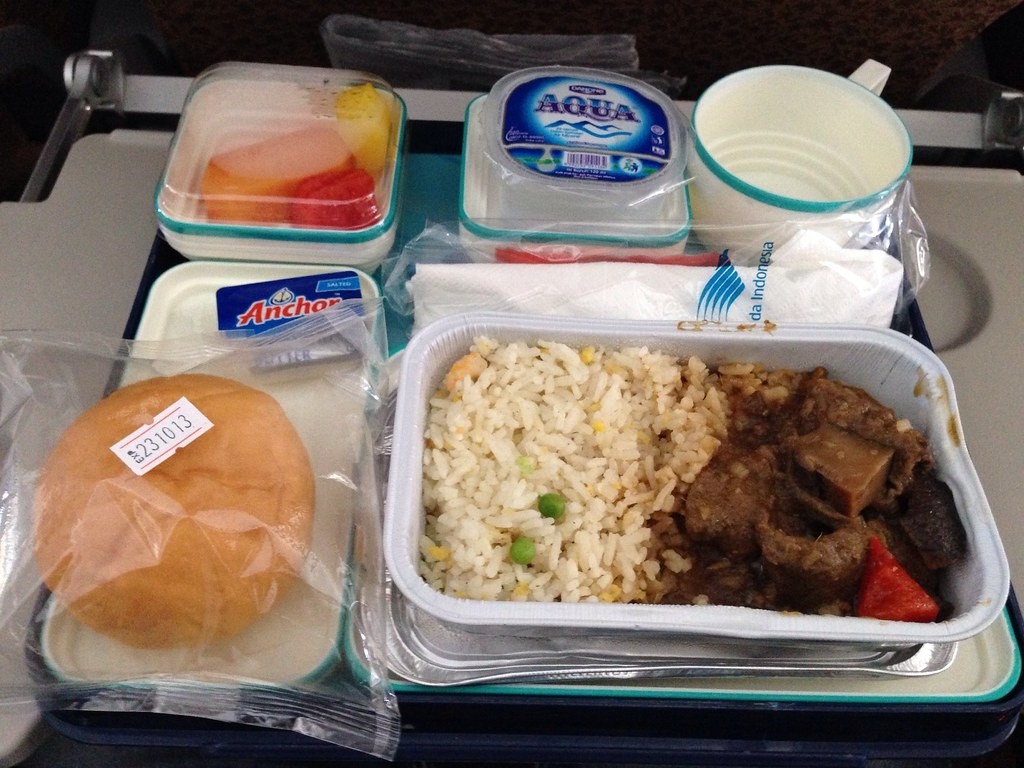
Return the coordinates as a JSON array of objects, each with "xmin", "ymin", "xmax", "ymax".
[
  {"xmin": 151, "ymin": 77, "xmax": 408, "ymax": 276},
  {"xmin": 458, "ymin": 94, "xmax": 693, "ymax": 262}
]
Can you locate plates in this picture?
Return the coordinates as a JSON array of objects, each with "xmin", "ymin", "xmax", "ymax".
[{"xmin": 41, "ymin": 257, "xmax": 383, "ymax": 693}]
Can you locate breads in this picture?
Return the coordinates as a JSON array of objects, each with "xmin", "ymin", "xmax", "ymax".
[{"xmin": 29, "ymin": 373, "xmax": 316, "ymax": 651}]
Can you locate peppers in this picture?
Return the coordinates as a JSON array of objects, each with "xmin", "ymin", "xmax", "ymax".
[{"xmin": 852, "ymin": 534, "xmax": 940, "ymax": 624}]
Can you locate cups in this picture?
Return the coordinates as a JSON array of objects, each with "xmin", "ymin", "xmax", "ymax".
[{"xmin": 686, "ymin": 58, "xmax": 914, "ymax": 267}]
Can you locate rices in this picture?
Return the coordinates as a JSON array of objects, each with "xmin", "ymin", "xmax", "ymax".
[{"xmin": 415, "ymin": 335, "xmax": 807, "ymax": 608}]
